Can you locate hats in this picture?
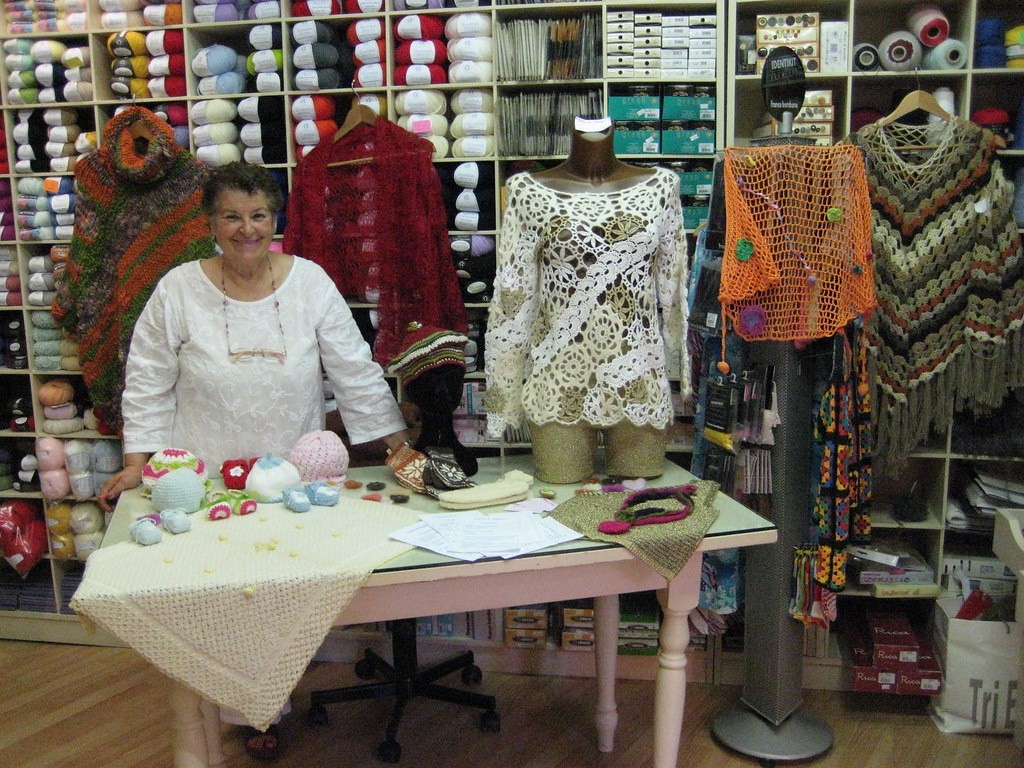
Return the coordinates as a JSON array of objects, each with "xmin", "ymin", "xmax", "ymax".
[
  {"xmin": 245, "ymin": 452, "xmax": 301, "ymax": 503},
  {"xmin": 385, "ymin": 320, "xmax": 469, "ymax": 387},
  {"xmin": 150, "ymin": 467, "xmax": 205, "ymax": 514},
  {"xmin": 288, "ymin": 430, "xmax": 349, "ymax": 483},
  {"xmin": 140, "ymin": 448, "xmax": 214, "ymax": 498}
]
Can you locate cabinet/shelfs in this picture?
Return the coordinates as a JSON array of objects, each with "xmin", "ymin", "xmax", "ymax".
[
  {"xmin": 714, "ymin": 0, "xmax": 1024, "ymax": 695},
  {"xmin": 0, "ymin": 0, "xmax": 726, "ymax": 687}
]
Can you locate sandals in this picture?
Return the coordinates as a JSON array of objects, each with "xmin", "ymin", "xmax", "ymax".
[{"xmin": 244, "ymin": 724, "xmax": 280, "ymax": 760}]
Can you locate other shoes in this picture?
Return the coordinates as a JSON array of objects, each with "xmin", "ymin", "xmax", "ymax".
[
  {"xmin": 305, "ymin": 482, "xmax": 340, "ymax": 506},
  {"xmin": 282, "ymin": 487, "xmax": 310, "ymax": 513},
  {"xmin": 159, "ymin": 508, "xmax": 190, "ymax": 533},
  {"xmin": 209, "ymin": 492, "xmax": 231, "ymax": 521},
  {"xmin": 227, "ymin": 489, "xmax": 257, "ymax": 515},
  {"xmin": 220, "ymin": 457, "xmax": 259, "ymax": 490},
  {"xmin": 128, "ymin": 518, "xmax": 161, "ymax": 544}
]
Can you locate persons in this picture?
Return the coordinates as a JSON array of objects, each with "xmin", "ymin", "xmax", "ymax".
[
  {"xmin": 484, "ymin": 116, "xmax": 688, "ymax": 482},
  {"xmin": 98, "ymin": 161, "xmax": 409, "ymax": 761}
]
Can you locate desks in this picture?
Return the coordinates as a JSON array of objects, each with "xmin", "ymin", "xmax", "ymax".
[{"xmin": 69, "ymin": 449, "xmax": 778, "ymax": 768}]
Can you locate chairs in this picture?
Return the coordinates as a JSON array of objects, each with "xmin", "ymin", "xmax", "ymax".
[{"xmin": 305, "ymin": 402, "xmax": 501, "ymax": 763}]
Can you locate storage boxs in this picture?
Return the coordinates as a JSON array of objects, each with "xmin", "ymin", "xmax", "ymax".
[
  {"xmin": 835, "ymin": 598, "xmax": 944, "ymax": 697},
  {"xmin": 616, "ymin": 598, "xmax": 660, "ymax": 656},
  {"xmin": 503, "ymin": 602, "xmax": 552, "ymax": 647},
  {"xmin": 620, "ymin": 160, "xmax": 714, "ymax": 229},
  {"xmin": 941, "ymin": 539, "xmax": 1018, "ymax": 593},
  {"xmin": 607, "ymin": 84, "xmax": 716, "ymax": 155},
  {"xmin": 858, "ymin": 549, "xmax": 940, "ymax": 598},
  {"xmin": 737, "ymin": 12, "xmax": 820, "ymax": 75},
  {"xmin": 606, "ymin": 12, "xmax": 716, "ymax": 79},
  {"xmin": 752, "ymin": 90, "xmax": 835, "ymax": 147},
  {"xmin": 551, "ymin": 599, "xmax": 595, "ymax": 651},
  {"xmin": 820, "ymin": 22, "xmax": 849, "ymax": 74}
]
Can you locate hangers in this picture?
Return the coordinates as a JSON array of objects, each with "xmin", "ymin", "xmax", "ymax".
[
  {"xmin": 294, "ymin": 80, "xmax": 437, "ymax": 174},
  {"xmin": 832, "ymin": 67, "xmax": 1006, "ymax": 150},
  {"xmin": 127, "ymin": 93, "xmax": 155, "ymax": 141}
]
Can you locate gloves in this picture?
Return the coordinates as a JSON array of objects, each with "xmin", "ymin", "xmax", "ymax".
[
  {"xmin": 385, "ymin": 442, "xmax": 431, "ymax": 493},
  {"xmin": 426, "ymin": 446, "xmax": 470, "ymax": 488}
]
